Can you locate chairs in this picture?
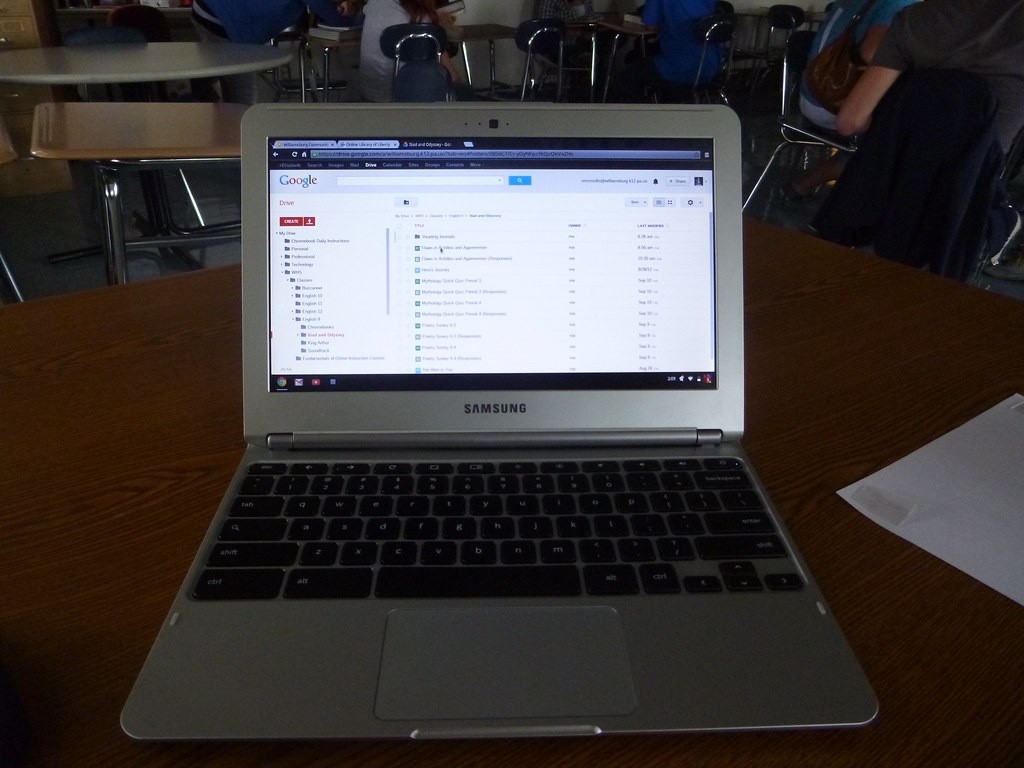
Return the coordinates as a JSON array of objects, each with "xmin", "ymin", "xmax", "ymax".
[
  {"xmin": 249, "ymin": 0, "xmax": 1022, "ymax": 279},
  {"xmin": 65, "ymin": 26, "xmax": 145, "ymax": 42},
  {"xmin": 109, "ymin": 4, "xmax": 172, "ymax": 42}
]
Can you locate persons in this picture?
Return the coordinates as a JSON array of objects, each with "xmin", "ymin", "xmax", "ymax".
[
  {"xmin": 536, "ymin": 0, "xmax": 594, "ymax": 103},
  {"xmin": 189, "ymin": 0, "xmax": 464, "ymax": 103},
  {"xmin": 796, "ymin": 0, "xmax": 1024, "ymax": 283},
  {"xmin": 613, "ymin": 0, "xmax": 722, "ymax": 103}
]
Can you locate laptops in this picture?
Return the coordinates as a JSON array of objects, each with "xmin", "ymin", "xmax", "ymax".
[{"xmin": 120, "ymin": 101, "xmax": 878, "ymax": 740}]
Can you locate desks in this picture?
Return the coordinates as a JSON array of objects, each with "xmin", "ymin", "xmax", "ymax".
[
  {"xmin": 0, "ymin": 121, "xmax": 24, "ymax": 304},
  {"xmin": 29, "ymin": 101, "xmax": 241, "ymax": 286},
  {"xmin": 0, "ymin": 218, "xmax": 1024, "ymax": 768},
  {"xmin": 0, "ymin": 42, "xmax": 293, "ymax": 270}
]
